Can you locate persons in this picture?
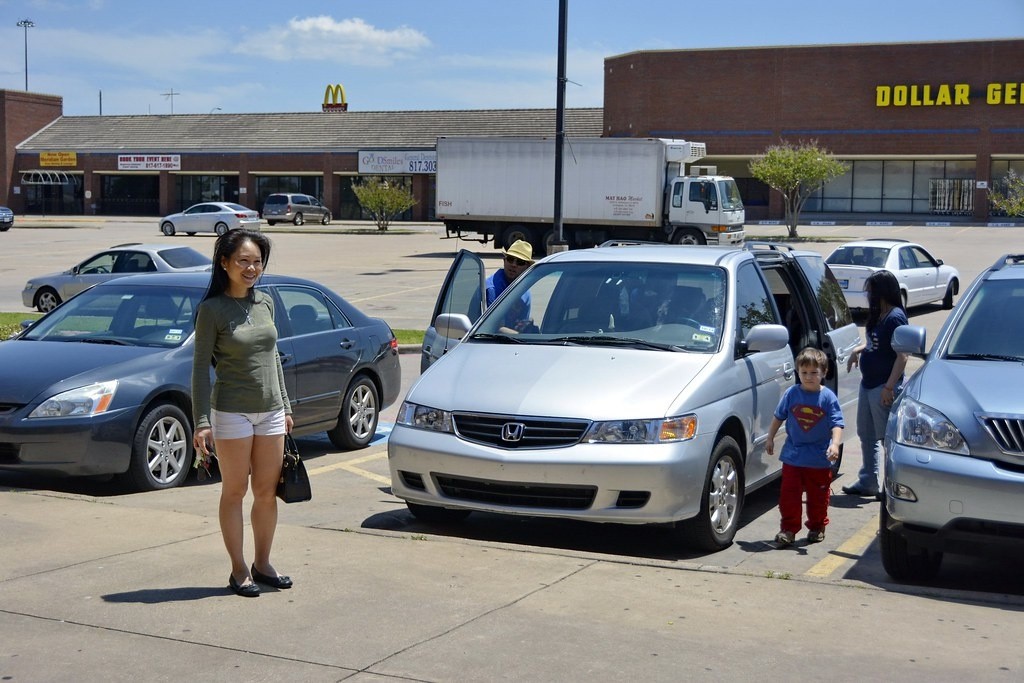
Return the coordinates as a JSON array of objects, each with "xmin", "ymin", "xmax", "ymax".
[
  {"xmin": 841, "ymin": 270, "xmax": 910, "ymax": 500},
  {"xmin": 764, "ymin": 347, "xmax": 845, "ymax": 546},
  {"xmin": 191, "ymin": 228, "xmax": 295, "ymax": 597},
  {"xmin": 862, "ymin": 248, "xmax": 874, "ymax": 261},
  {"xmin": 459, "ymin": 239, "xmax": 536, "ymax": 337}
]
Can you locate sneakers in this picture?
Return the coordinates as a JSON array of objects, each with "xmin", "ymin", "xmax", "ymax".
[
  {"xmin": 776, "ymin": 531, "xmax": 796, "ymax": 543},
  {"xmin": 808, "ymin": 530, "xmax": 826, "ymax": 541}
]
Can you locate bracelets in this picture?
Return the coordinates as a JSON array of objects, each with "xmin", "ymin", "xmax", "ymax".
[{"xmin": 883, "ymin": 383, "xmax": 893, "ymax": 391}]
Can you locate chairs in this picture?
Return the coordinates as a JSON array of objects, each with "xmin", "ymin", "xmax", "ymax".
[
  {"xmin": 675, "ymin": 286, "xmax": 706, "ymax": 324},
  {"xmin": 772, "ymin": 293, "xmax": 795, "ymax": 338},
  {"xmin": 852, "ymin": 255, "xmax": 865, "ymax": 264},
  {"xmin": 290, "ymin": 305, "xmax": 318, "ymax": 330},
  {"xmin": 122, "ymin": 259, "xmax": 139, "ymax": 271}
]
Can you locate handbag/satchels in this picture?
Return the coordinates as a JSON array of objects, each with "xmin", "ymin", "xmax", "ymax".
[{"xmin": 276, "ymin": 432, "xmax": 312, "ymax": 503}]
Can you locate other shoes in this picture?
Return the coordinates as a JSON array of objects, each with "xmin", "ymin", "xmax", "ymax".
[{"xmin": 841, "ymin": 485, "xmax": 876, "ymax": 497}]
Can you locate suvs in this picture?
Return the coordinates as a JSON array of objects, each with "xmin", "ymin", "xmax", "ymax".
[
  {"xmin": 262, "ymin": 193, "xmax": 333, "ymax": 226},
  {"xmin": 388, "ymin": 239, "xmax": 865, "ymax": 553},
  {"xmin": 879, "ymin": 254, "xmax": 1024, "ymax": 581}
]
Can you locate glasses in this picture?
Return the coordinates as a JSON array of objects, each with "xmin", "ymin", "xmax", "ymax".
[
  {"xmin": 230, "ymin": 255, "xmax": 263, "ymax": 268},
  {"xmin": 505, "ymin": 254, "xmax": 526, "ymax": 267}
]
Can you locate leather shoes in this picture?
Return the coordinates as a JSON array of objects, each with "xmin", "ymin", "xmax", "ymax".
[
  {"xmin": 228, "ymin": 573, "xmax": 260, "ymax": 597},
  {"xmin": 250, "ymin": 562, "xmax": 294, "ymax": 588}
]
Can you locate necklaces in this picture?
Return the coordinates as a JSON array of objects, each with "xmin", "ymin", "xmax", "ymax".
[{"xmin": 229, "ymin": 292, "xmax": 250, "ymax": 325}]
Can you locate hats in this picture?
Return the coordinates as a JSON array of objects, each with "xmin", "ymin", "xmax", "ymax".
[{"xmin": 502, "ymin": 240, "xmax": 536, "ymax": 264}]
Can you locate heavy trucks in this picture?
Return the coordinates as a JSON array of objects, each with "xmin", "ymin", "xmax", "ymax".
[{"xmin": 436, "ymin": 137, "xmax": 746, "ymax": 260}]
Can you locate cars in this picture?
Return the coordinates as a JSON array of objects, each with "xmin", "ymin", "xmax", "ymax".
[
  {"xmin": 22, "ymin": 240, "xmax": 216, "ymax": 321},
  {"xmin": 0, "ymin": 206, "xmax": 14, "ymax": 232},
  {"xmin": 823, "ymin": 238, "xmax": 959, "ymax": 315},
  {"xmin": 0, "ymin": 272, "xmax": 401, "ymax": 490},
  {"xmin": 158, "ymin": 202, "xmax": 262, "ymax": 237}
]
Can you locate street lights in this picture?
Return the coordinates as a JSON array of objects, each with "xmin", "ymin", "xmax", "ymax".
[{"xmin": 16, "ymin": 19, "xmax": 36, "ymax": 91}]
